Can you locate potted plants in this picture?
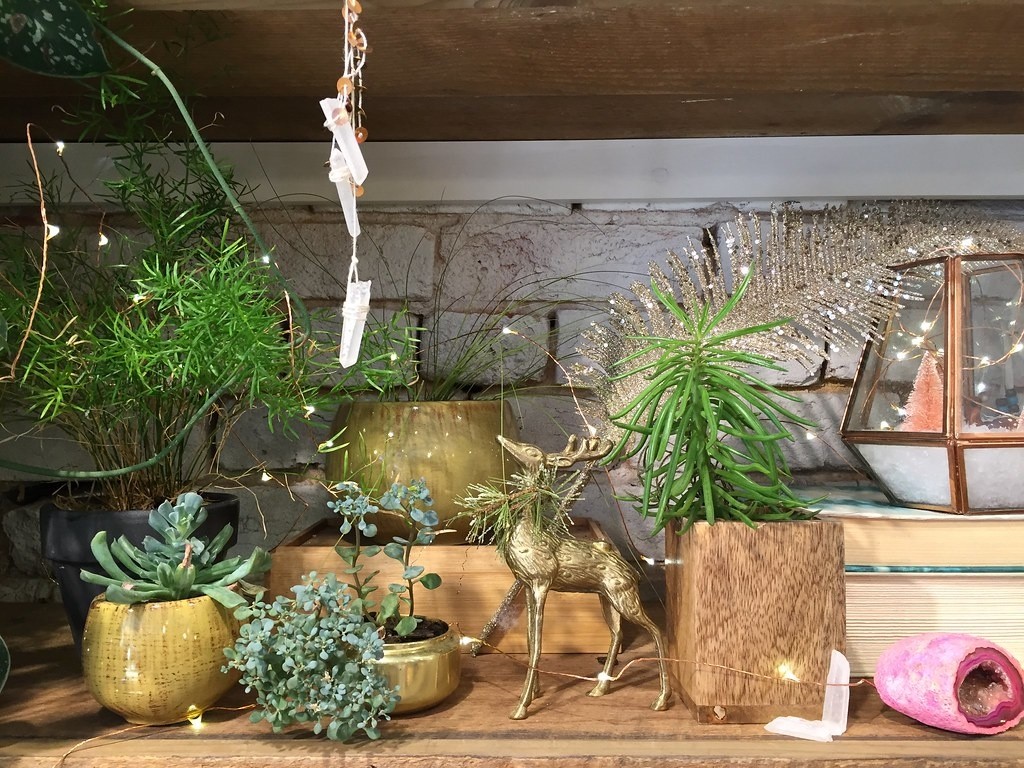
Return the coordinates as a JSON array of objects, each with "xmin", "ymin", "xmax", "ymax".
[
  {"xmin": 78, "ymin": 492, "xmax": 273, "ymax": 728},
  {"xmin": 0, "ymin": 0, "xmax": 430, "ymax": 653},
  {"xmin": 220, "ymin": 478, "xmax": 461, "ymax": 744},
  {"xmin": 323, "ymin": 194, "xmax": 676, "ymax": 546},
  {"xmin": 597, "ymin": 257, "xmax": 847, "ymax": 727}
]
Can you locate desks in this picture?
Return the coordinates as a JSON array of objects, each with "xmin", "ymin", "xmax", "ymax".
[{"xmin": 0, "ymin": 599, "xmax": 1024, "ymax": 768}]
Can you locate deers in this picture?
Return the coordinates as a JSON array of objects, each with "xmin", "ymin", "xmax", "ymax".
[{"xmin": 470, "ymin": 435, "xmax": 672, "ymax": 719}]
